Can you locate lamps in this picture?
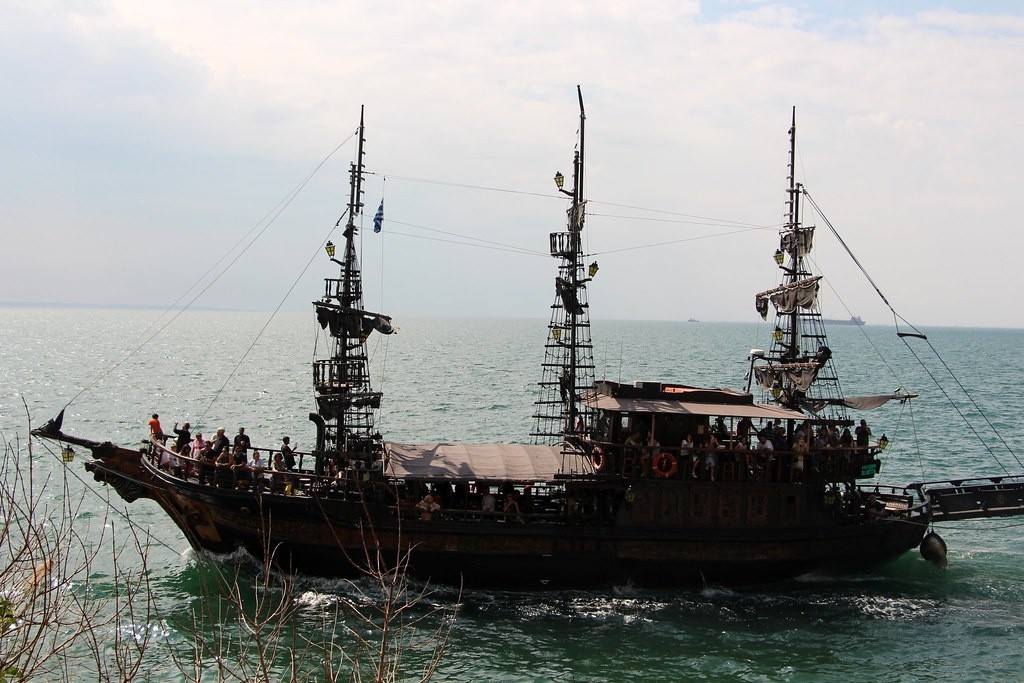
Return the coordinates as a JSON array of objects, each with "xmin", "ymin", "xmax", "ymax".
[
  {"xmin": 624, "ymin": 489, "xmax": 634, "ymax": 503},
  {"xmin": 825, "ymin": 489, "xmax": 837, "ymax": 508},
  {"xmin": 589, "ymin": 261, "xmax": 599, "ymax": 278},
  {"xmin": 771, "ymin": 326, "xmax": 783, "ymax": 342},
  {"xmin": 552, "ymin": 324, "xmax": 561, "ymax": 342},
  {"xmin": 63, "ymin": 445, "xmax": 74, "ymax": 462},
  {"xmin": 769, "ymin": 382, "xmax": 780, "ymax": 398},
  {"xmin": 358, "ymin": 465, "xmax": 370, "ymax": 481},
  {"xmin": 876, "ymin": 433, "xmax": 888, "ymax": 451},
  {"xmin": 326, "ymin": 240, "xmax": 335, "ymax": 258},
  {"xmin": 554, "ymin": 171, "xmax": 563, "ymax": 188},
  {"xmin": 774, "ymin": 248, "xmax": 784, "ymax": 265}
]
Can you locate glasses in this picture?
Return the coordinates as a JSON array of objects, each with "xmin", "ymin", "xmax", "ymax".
[{"xmin": 507, "ymin": 496, "xmax": 512, "ymax": 498}]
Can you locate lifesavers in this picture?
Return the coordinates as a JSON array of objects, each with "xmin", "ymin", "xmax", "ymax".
[
  {"xmin": 590, "ymin": 447, "xmax": 605, "ymax": 470},
  {"xmin": 652, "ymin": 453, "xmax": 678, "ymax": 478}
]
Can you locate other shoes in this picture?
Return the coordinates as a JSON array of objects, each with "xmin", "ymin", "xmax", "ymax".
[
  {"xmin": 747, "ymin": 465, "xmax": 753, "ymax": 468},
  {"xmin": 711, "ymin": 477, "xmax": 714, "ymax": 481},
  {"xmin": 692, "ymin": 473, "xmax": 697, "ymax": 478}
]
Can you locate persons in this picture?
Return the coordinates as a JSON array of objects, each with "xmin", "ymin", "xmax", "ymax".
[
  {"xmin": 404, "ymin": 482, "xmax": 537, "ymax": 528},
  {"xmin": 628, "ymin": 420, "xmax": 887, "ymax": 527},
  {"xmin": 148, "ymin": 414, "xmax": 346, "ymax": 495}
]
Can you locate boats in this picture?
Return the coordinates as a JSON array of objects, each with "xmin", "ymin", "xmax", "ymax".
[{"xmin": 803, "ymin": 317, "xmax": 866, "ymax": 326}]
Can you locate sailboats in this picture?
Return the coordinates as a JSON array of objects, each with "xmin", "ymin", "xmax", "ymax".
[{"xmin": 29, "ymin": 86, "xmax": 951, "ymax": 586}]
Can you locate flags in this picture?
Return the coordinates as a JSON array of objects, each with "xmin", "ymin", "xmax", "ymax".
[{"xmin": 374, "ymin": 199, "xmax": 384, "ymax": 232}]
